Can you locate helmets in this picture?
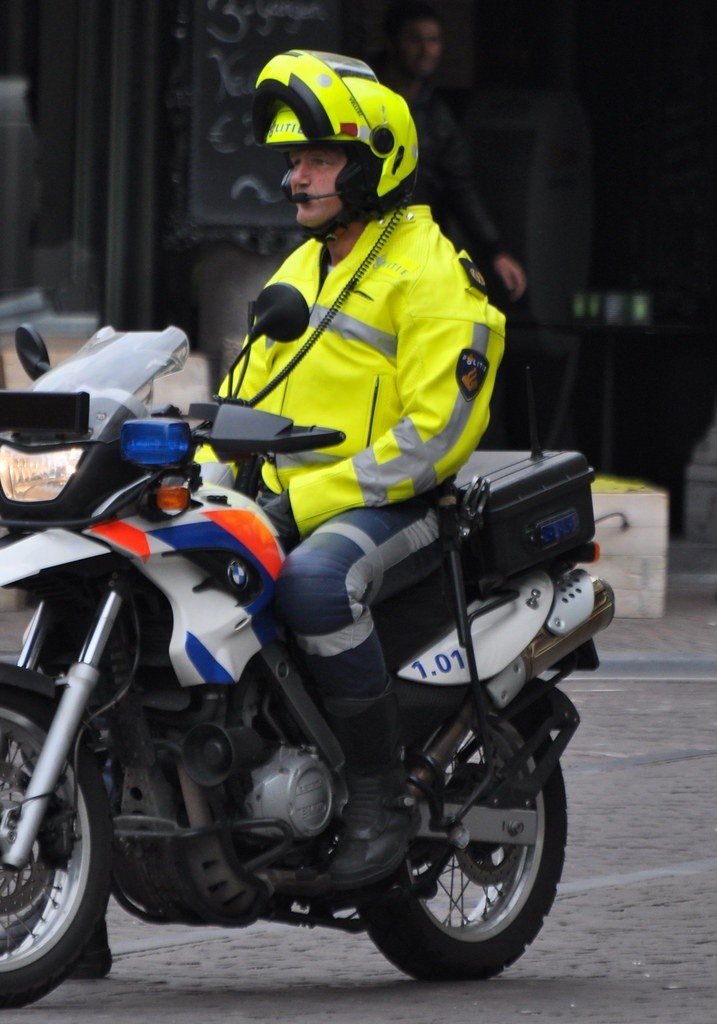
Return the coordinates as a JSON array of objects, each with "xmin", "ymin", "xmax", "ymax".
[{"xmin": 249, "ymin": 49, "xmax": 418, "ymax": 224}]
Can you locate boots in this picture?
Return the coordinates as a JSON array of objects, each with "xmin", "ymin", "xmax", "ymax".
[{"xmin": 312, "ymin": 671, "xmax": 422, "ymax": 889}]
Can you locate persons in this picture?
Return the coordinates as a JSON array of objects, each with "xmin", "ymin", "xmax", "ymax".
[
  {"xmin": 193, "ymin": 46, "xmax": 509, "ymax": 890},
  {"xmin": 371, "ymin": 1, "xmax": 529, "ymax": 303}
]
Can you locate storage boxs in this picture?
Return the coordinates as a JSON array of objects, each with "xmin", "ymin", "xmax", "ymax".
[{"xmin": 577, "ymin": 486, "xmax": 670, "ymax": 619}]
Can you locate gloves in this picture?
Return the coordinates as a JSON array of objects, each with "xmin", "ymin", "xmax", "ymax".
[{"xmin": 260, "ymin": 488, "xmax": 300, "ymax": 554}]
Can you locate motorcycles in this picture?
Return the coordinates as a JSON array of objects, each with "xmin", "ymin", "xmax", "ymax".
[{"xmin": 0, "ymin": 280, "xmax": 617, "ymax": 1010}]
